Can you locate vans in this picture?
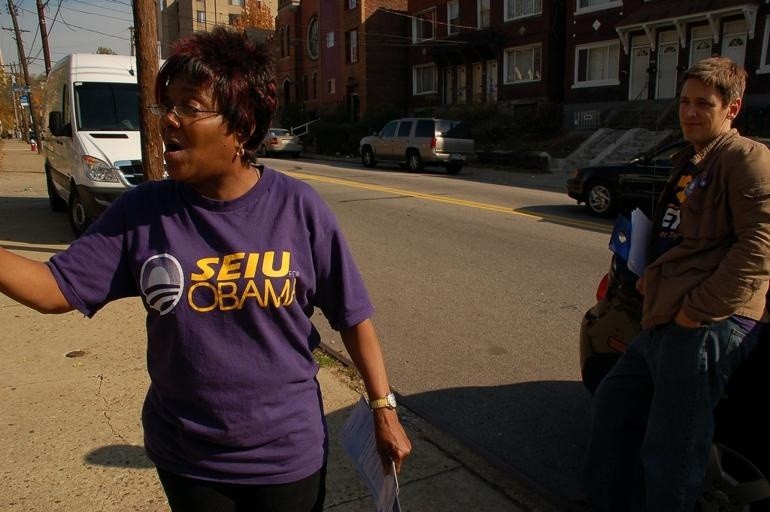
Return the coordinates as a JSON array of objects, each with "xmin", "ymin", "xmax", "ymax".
[{"xmin": 45, "ymin": 53, "xmax": 170, "ymax": 239}]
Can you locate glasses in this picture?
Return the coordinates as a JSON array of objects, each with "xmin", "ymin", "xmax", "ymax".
[{"xmin": 144, "ymin": 104, "xmax": 222, "ymax": 120}]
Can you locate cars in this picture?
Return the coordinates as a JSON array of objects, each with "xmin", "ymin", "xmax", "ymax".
[
  {"xmin": 256, "ymin": 127, "xmax": 304, "ymax": 157},
  {"xmin": 580, "ymin": 230, "xmax": 770, "ymax": 512},
  {"xmin": 567, "ymin": 139, "xmax": 692, "ymax": 216},
  {"xmin": 360, "ymin": 117, "xmax": 478, "ymax": 173}
]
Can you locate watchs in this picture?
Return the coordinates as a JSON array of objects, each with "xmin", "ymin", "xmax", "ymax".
[{"xmin": 368, "ymin": 391, "xmax": 398, "ymax": 411}]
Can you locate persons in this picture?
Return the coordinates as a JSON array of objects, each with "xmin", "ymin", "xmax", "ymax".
[
  {"xmin": 1, "ymin": 26, "xmax": 413, "ymax": 512},
  {"xmin": 593, "ymin": 57, "xmax": 770, "ymax": 511}
]
